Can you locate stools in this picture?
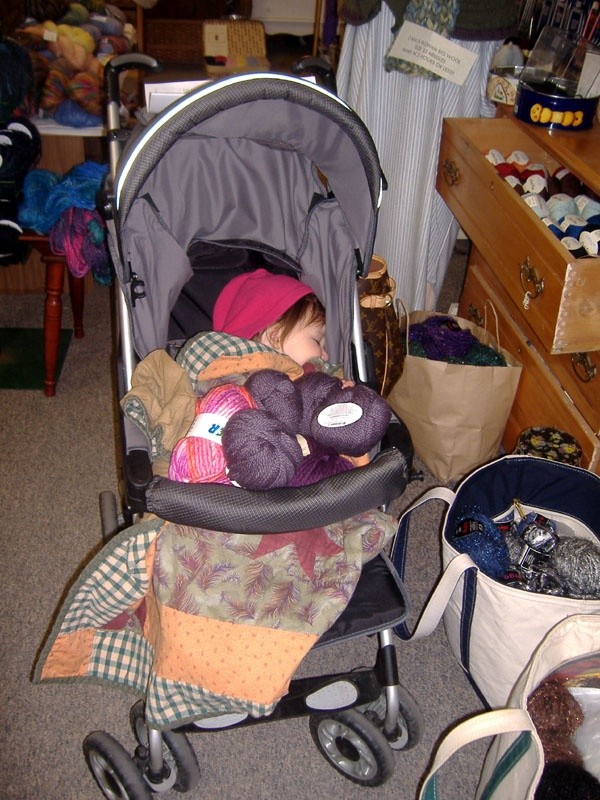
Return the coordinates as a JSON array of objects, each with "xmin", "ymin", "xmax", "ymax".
[{"xmin": 19, "ymin": 228, "xmax": 84, "ymax": 396}]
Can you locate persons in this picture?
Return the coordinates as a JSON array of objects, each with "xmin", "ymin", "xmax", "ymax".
[
  {"xmin": 335, "ymin": 0, "xmax": 519, "ymax": 312},
  {"xmin": 147, "ymin": 267, "xmax": 392, "ymax": 730}
]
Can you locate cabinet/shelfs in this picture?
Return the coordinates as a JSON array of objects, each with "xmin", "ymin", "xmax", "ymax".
[{"xmin": 435, "ymin": 102, "xmax": 600, "ymax": 477}]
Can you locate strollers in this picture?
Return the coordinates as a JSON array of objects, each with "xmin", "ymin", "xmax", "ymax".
[{"xmin": 79, "ymin": 53, "xmax": 425, "ymax": 800}]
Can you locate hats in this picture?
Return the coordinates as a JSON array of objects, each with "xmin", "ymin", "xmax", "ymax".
[{"xmin": 211, "ymin": 269, "xmax": 316, "ymax": 342}]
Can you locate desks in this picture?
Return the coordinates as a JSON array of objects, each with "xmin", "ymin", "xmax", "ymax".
[{"xmin": 0, "ymin": 113, "xmax": 108, "ymax": 295}]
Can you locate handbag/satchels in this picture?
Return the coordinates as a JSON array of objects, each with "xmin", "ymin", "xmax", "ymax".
[
  {"xmin": 392, "ymin": 454, "xmax": 600, "ymax": 710},
  {"xmin": 386, "ymin": 298, "xmax": 524, "ymax": 484},
  {"xmin": 414, "ymin": 613, "xmax": 600, "ymax": 800}
]
